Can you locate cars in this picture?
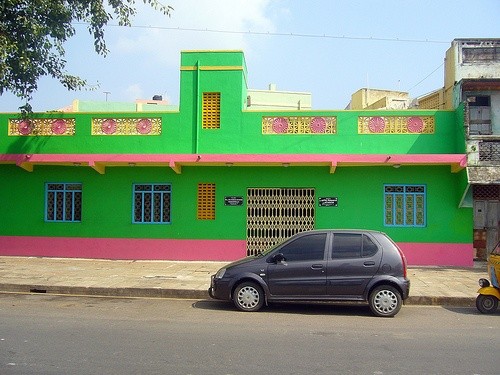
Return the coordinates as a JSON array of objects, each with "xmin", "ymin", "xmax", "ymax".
[{"xmin": 208, "ymin": 229, "xmax": 411, "ymax": 317}]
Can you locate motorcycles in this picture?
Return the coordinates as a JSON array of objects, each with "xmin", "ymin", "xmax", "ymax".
[{"xmin": 475, "ymin": 240, "xmax": 500, "ymax": 314}]
